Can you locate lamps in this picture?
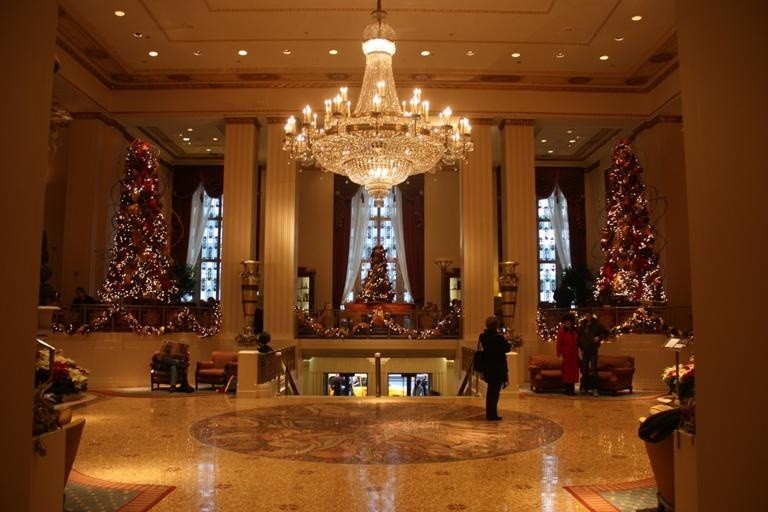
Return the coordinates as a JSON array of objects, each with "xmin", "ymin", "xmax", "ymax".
[{"xmin": 281, "ymin": 0, "xmax": 474, "ymax": 207}]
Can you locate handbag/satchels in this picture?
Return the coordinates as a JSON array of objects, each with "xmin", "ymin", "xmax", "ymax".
[{"xmin": 474, "ymin": 351, "xmax": 499, "ymax": 371}]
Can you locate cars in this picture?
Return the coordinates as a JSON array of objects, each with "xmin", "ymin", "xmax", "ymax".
[{"xmin": 328, "ymin": 374, "xmax": 407, "ymax": 397}]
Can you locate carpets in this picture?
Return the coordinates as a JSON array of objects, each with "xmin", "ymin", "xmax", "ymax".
[
  {"xmin": 92, "ymin": 386, "xmax": 235, "ymax": 398},
  {"xmin": 64, "ymin": 468, "xmax": 177, "ymax": 512},
  {"xmin": 521, "ymin": 389, "xmax": 662, "ymax": 400},
  {"xmin": 564, "ymin": 478, "xmax": 658, "ymax": 512}
]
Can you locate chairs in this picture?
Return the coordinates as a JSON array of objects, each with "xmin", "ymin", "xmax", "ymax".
[
  {"xmin": 195, "ymin": 352, "xmax": 238, "ymax": 390},
  {"xmin": 528, "ymin": 353, "xmax": 563, "ymax": 393},
  {"xmin": 149, "ymin": 351, "xmax": 190, "ymax": 390},
  {"xmin": 580, "ymin": 355, "xmax": 635, "ymax": 392}
]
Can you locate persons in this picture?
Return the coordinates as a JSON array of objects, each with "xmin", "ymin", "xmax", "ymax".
[
  {"xmin": 71, "ymin": 287, "xmax": 96, "ymax": 313},
  {"xmin": 423, "ymin": 376, "xmax": 427, "ymax": 395},
  {"xmin": 478, "ymin": 316, "xmax": 511, "ymax": 420},
  {"xmin": 577, "ymin": 312, "xmax": 609, "ymax": 397},
  {"xmin": 152, "ymin": 350, "xmax": 184, "ymax": 393},
  {"xmin": 556, "ymin": 313, "xmax": 580, "ymax": 396},
  {"xmin": 415, "ymin": 380, "xmax": 424, "ymax": 396}
]
